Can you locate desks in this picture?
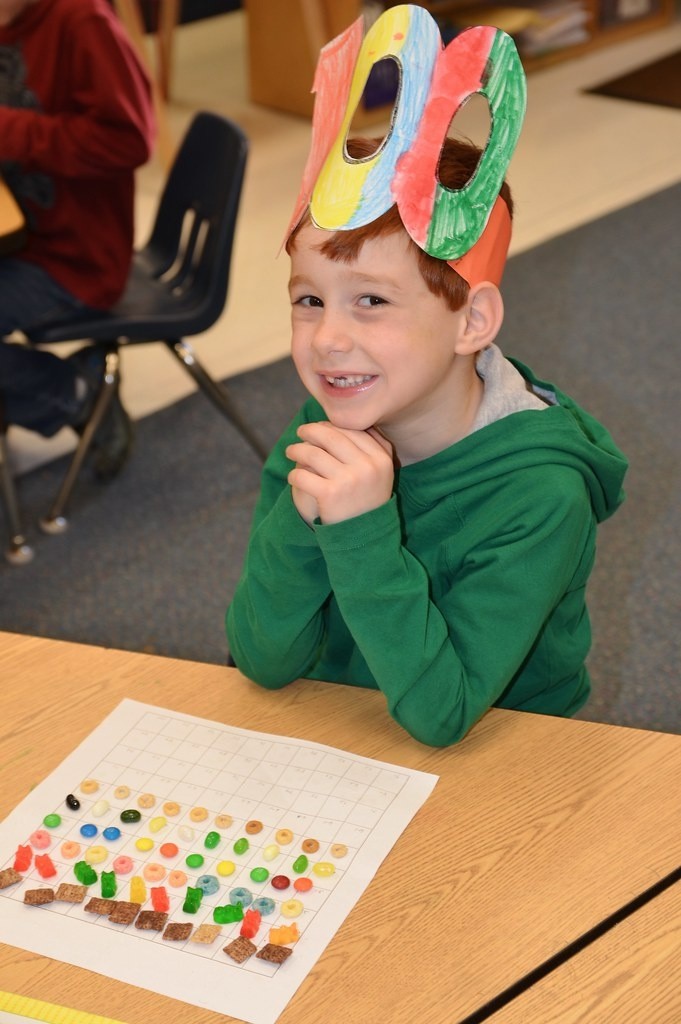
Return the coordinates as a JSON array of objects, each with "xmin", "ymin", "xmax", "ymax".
[
  {"xmin": 478, "ymin": 877, "xmax": 681, "ymax": 1024},
  {"xmin": 2, "ymin": 627, "xmax": 681, "ymax": 1024}
]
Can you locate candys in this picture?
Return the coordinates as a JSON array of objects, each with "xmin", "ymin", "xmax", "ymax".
[{"xmin": 0, "ymin": 780, "xmax": 347, "ymax": 964}]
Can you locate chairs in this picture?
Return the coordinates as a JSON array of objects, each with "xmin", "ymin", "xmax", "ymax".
[{"xmin": 16, "ymin": 110, "xmax": 281, "ymax": 538}]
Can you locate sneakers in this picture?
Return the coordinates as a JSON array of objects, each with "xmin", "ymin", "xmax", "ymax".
[{"xmin": 65, "ymin": 345, "xmax": 134, "ymax": 464}]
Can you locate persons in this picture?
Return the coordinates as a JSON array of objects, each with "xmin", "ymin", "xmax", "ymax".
[
  {"xmin": 0, "ymin": 0, "xmax": 160, "ymax": 484},
  {"xmin": 225, "ymin": 132, "xmax": 630, "ymax": 755}
]
